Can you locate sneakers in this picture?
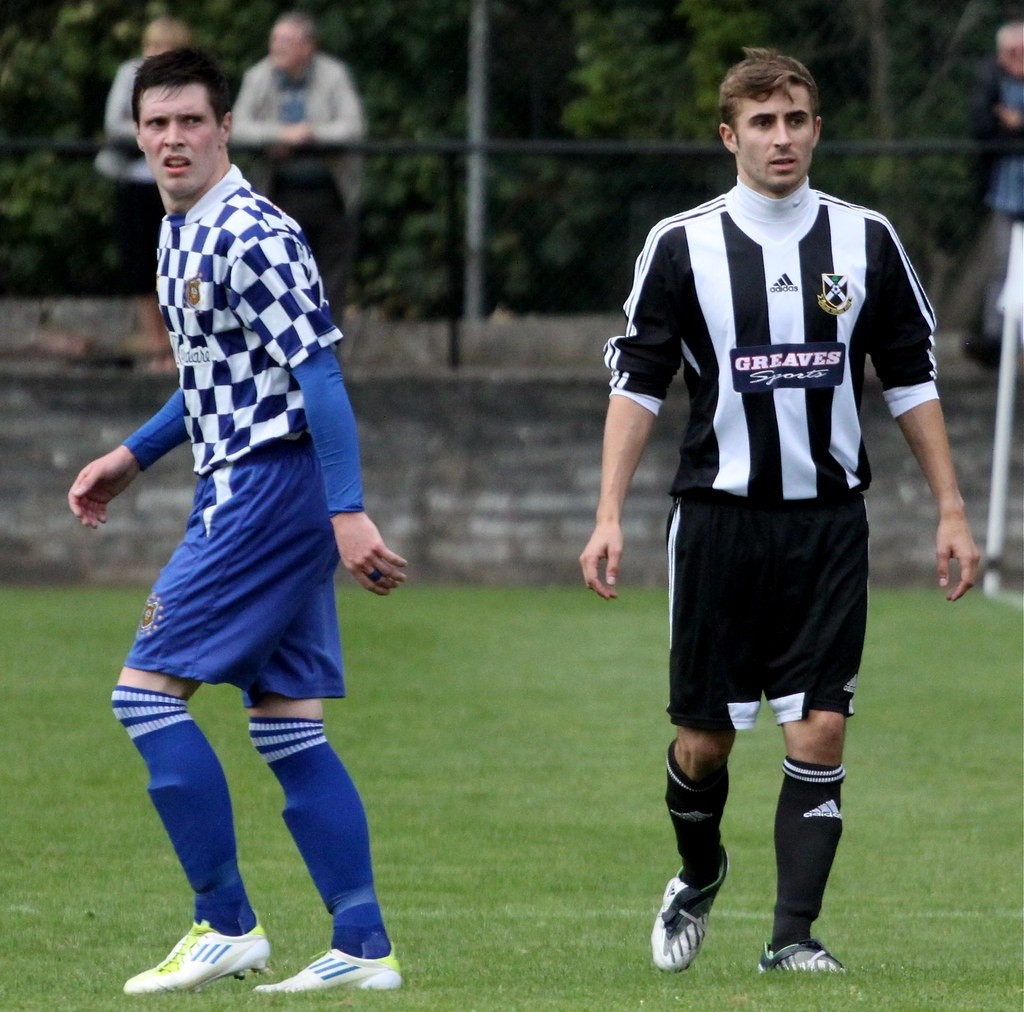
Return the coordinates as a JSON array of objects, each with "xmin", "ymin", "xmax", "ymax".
[
  {"xmin": 650, "ymin": 844, "xmax": 729, "ymax": 972},
  {"xmin": 123, "ymin": 914, "xmax": 273, "ymax": 995},
  {"xmin": 757, "ymin": 939, "xmax": 843, "ymax": 974},
  {"xmin": 252, "ymin": 940, "xmax": 404, "ymax": 994}
]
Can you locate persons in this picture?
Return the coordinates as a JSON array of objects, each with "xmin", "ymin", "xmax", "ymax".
[
  {"xmin": 961, "ymin": 23, "xmax": 1023, "ymax": 365},
  {"xmin": 99, "ymin": 21, "xmax": 191, "ymax": 300},
  {"xmin": 579, "ymin": 41, "xmax": 979, "ymax": 978},
  {"xmin": 65, "ymin": 45, "xmax": 407, "ymax": 1001},
  {"xmin": 229, "ymin": 14, "xmax": 369, "ymax": 316}
]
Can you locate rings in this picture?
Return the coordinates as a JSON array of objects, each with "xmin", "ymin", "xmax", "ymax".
[{"xmin": 369, "ymin": 569, "xmax": 383, "ymax": 581}]
